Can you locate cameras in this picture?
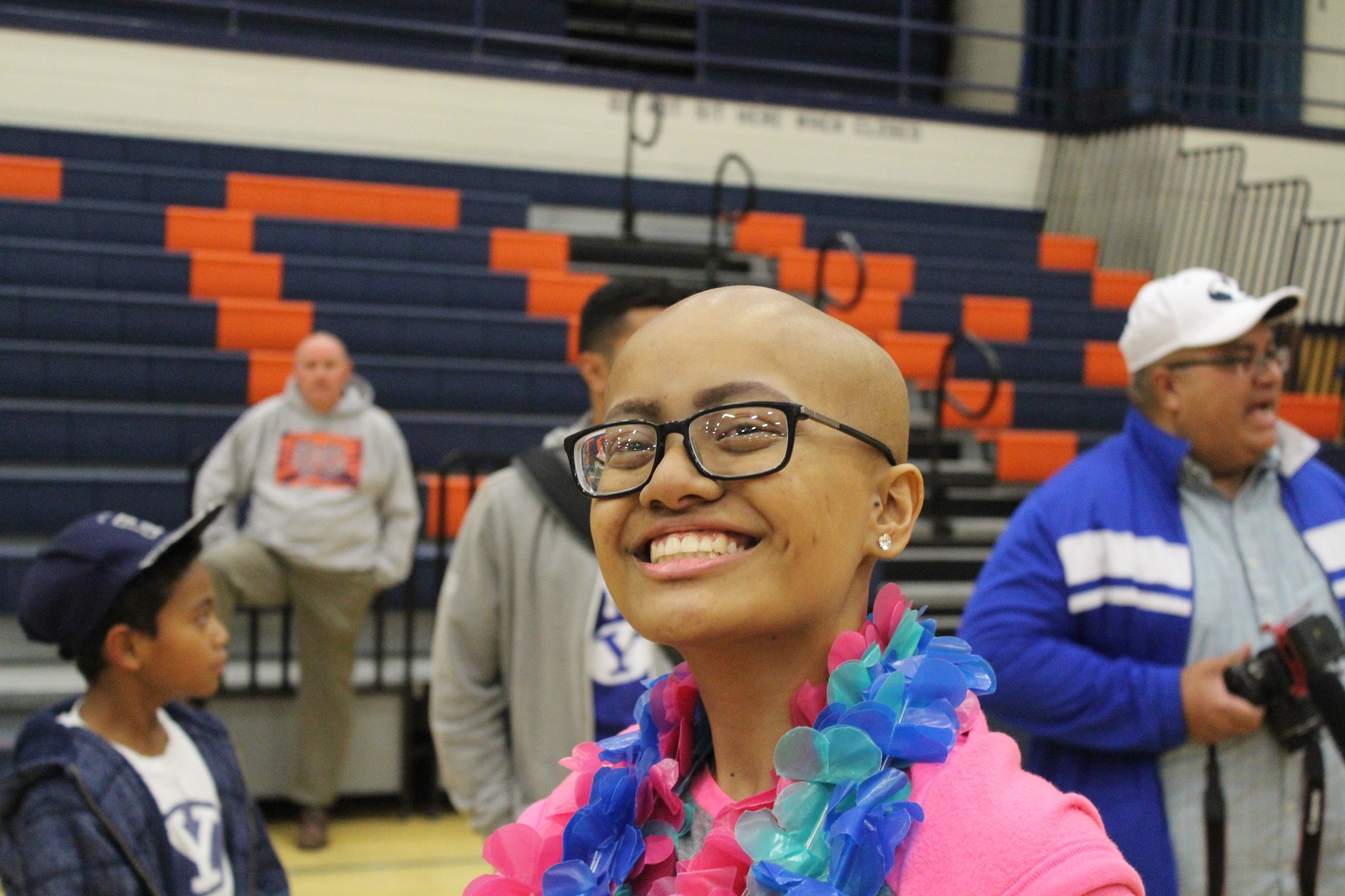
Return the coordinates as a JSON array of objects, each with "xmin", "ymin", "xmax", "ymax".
[{"xmin": 1222, "ymin": 613, "xmax": 1344, "ymax": 748}]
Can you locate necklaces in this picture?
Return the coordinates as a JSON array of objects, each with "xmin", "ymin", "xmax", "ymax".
[{"xmin": 535, "ymin": 581, "xmax": 1001, "ymax": 896}]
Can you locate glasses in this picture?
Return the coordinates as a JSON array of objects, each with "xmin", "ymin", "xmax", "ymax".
[
  {"xmin": 1169, "ymin": 346, "xmax": 1290, "ymax": 376},
  {"xmin": 563, "ymin": 401, "xmax": 897, "ymax": 501}
]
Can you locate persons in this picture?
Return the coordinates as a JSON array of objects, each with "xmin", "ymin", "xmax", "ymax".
[
  {"xmin": 0, "ymin": 499, "xmax": 290, "ymax": 896},
  {"xmin": 461, "ymin": 285, "xmax": 1145, "ymax": 896},
  {"xmin": 428, "ymin": 278, "xmax": 691, "ymax": 838},
  {"xmin": 193, "ymin": 334, "xmax": 421, "ymax": 854},
  {"xmin": 951, "ymin": 267, "xmax": 1345, "ymax": 896}
]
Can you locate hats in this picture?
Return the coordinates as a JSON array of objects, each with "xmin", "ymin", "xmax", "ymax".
[
  {"xmin": 17, "ymin": 496, "xmax": 225, "ymax": 663},
  {"xmin": 1116, "ymin": 268, "xmax": 1301, "ymax": 375}
]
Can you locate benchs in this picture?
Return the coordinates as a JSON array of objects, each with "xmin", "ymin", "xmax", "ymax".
[{"xmin": 0, "ymin": 124, "xmax": 1345, "ymax": 620}]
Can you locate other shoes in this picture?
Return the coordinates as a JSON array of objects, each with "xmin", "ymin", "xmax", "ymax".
[{"xmin": 298, "ymin": 810, "xmax": 326, "ymax": 848}]
